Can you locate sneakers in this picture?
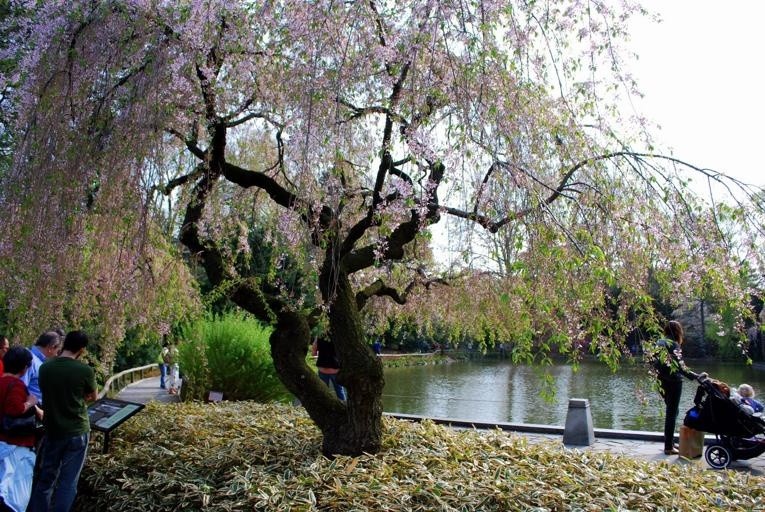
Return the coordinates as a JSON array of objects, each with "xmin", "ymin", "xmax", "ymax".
[{"xmin": 665, "ymin": 443, "xmax": 679, "ymax": 454}]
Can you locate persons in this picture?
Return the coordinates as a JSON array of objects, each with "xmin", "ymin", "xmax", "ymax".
[
  {"xmin": 0, "ymin": 328, "xmax": 99, "ymax": 512},
  {"xmin": 737, "ymin": 384, "xmax": 764, "ymax": 413},
  {"xmin": 312, "ymin": 330, "xmax": 346, "ymax": 402},
  {"xmin": 158, "ymin": 343, "xmax": 170, "ymax": 390},
  {"xmin": 167, "ymin": 337, "xmax": 180, "ymax": 394},
  {"xmin": 652, "ymin": 320, "xmax": 708, "ymax": 454}
]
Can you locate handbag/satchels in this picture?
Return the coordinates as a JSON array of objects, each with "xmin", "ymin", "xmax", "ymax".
[
  {"xmin": 679, "ymin": 425, "xmax": 704, "ymax": 458},
  {"xmin": 0, "ymin": 407, "xmax": 37, "ymax": 437}
]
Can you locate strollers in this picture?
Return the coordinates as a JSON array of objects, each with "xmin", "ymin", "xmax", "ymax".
[{"xmin": 693, "ymin": 370, "xmax": 765, "ymax": 469}]
